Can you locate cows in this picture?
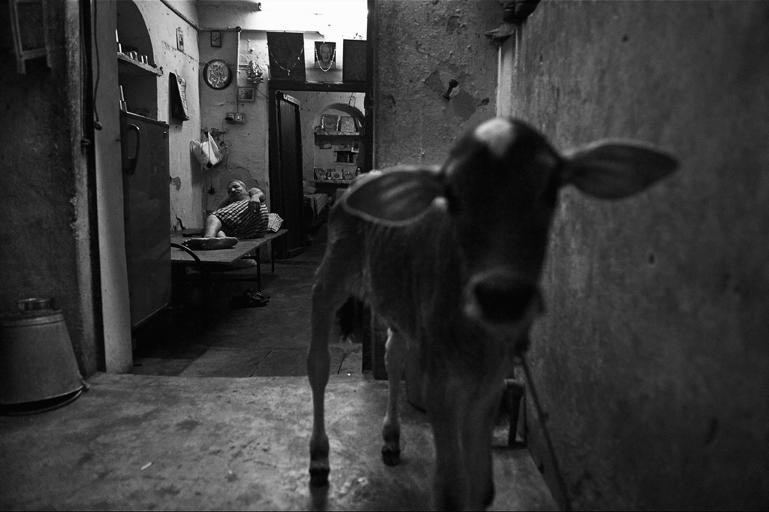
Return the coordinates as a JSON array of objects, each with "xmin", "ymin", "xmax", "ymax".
[{"xmin": 306, "ymin": 117, "xmax": 683, "ymax": 511}]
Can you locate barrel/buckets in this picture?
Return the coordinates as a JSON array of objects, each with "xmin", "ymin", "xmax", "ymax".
[{"xmin": 0, "ymin": 309, "xmax": 90, "ymax": 416}]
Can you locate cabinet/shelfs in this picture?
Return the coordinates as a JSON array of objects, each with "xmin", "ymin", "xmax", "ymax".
[{"xmin": 313, "ymin": 129, "xmax": 361, "ymax": 184}]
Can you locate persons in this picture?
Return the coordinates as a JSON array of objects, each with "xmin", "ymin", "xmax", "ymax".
[{"xmin": 182, "ymin": 178, "xmax": 269, "ymax": 253}]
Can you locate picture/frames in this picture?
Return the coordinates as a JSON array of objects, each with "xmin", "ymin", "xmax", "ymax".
[
  {"xmin": 237, "ymin": 87, "xmax": 255, "ymax": 102},
  {"xmin": 210, "ymin": 30, "xmax": 222, "ymax": 48}
]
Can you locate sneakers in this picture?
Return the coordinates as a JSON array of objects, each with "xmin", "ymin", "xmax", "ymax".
[
  {"xmin": 232, "ymin": 295, "xmax": 269, "ymax": 307},
  {"xmin": 243, "ymin": 287, "xmax": 270, "ymax": 299}
]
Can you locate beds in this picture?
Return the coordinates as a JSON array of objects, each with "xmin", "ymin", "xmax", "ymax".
[{"xmin": 170, "ymin": 226, "xmax": 289, "ymax": 323}]
